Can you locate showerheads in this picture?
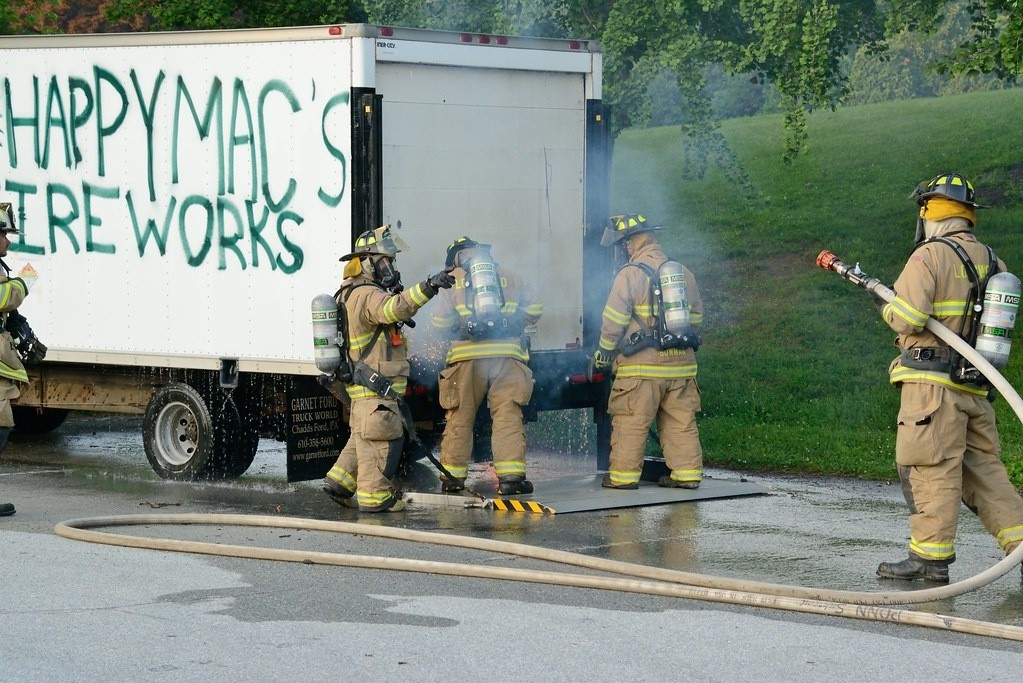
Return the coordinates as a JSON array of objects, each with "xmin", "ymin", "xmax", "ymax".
[{"xmin": 816, "ymin": 250, "xmax": 880, "ymax": 292}]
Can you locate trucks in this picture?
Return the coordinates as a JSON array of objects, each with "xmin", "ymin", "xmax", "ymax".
[{"xmin": 0, "ymin": 18, "xmax": 609, "ymax": 483}]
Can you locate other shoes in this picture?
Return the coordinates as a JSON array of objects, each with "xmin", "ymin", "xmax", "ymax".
[{"xmin": 0, "ymin": 503, "xmax": 17, "ymax": 515}]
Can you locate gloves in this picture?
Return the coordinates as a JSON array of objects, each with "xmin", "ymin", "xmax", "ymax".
[
  {"xmin": 428, "ymin": 266, "xmax": 455, "ymax": 289},
  {"xmin": 7, "ymin": 276, "xmax": 29, "ymax": 298},
  {"xmin": 866, "ymin": 278, "xmax": 897, "ymax": 313},
  {"xmin": 590, "ymin": 348, "xmax": 614, "ymax": 369}
]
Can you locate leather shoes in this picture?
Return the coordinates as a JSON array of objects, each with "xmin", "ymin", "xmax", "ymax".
[{"xmin": 875, "ymin": 557, "xmax": 949, "ymax": 582}]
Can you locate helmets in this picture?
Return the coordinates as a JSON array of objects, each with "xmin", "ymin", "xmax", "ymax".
[
  {"xmin": 445, "ymin": 236, "xmax": 492, "ymax": 267},
  {"xmin": 339, "ymin": 230, "xmax": 401, "ymax": 261},
  {"xmin": 0, "ymin": 209, "xmax": 16, "ymax": 231},
  {"xmin": 914, "ymin": 170, "xmax": 992, "ymax": 210},
  {"xmin": 612, "ymin": 214, "xmax": 665, "ymax": 241}
]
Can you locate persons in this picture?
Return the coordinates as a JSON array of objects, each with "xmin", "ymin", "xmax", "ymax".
[
  {"xmin": 594, "ymin": 214, "xmax": 702, "ymax": 488},
  {"xmin": 430, "ymin": 237, "xmax": 544, "ymax": 495},
  {"xmin": 322, "ymin": 231, "xmax": 456, "ymax": 512},
  {"xmin": 0, "ymin": 208, "xmax": 37, "ymax": 516},
  {"xmin": 876, "ymin": 172, "xmax": 1023, "ymax": 581}
]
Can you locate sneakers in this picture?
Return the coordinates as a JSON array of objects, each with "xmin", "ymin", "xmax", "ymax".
[
  {"xmin": 359, "ymin": 499, "xmax": 404, "ymax": 512},
  {"xmin": 658, "ymin": 475, "xmax": 701, "ymax": 489},
  {"xmin": 442, "ymin": 482, "xmax": 464, "ymax": 491},
  {"xmin": 498, "ymin": 480, "xmax": 533, "ymax": 495},
  {"xmin": 602, "ymin": 475, "xmax": 641, "ymax": 489},
  {"xmin": 323, "ymin": 482, "xmax": 357, "ymax": 507}
]
